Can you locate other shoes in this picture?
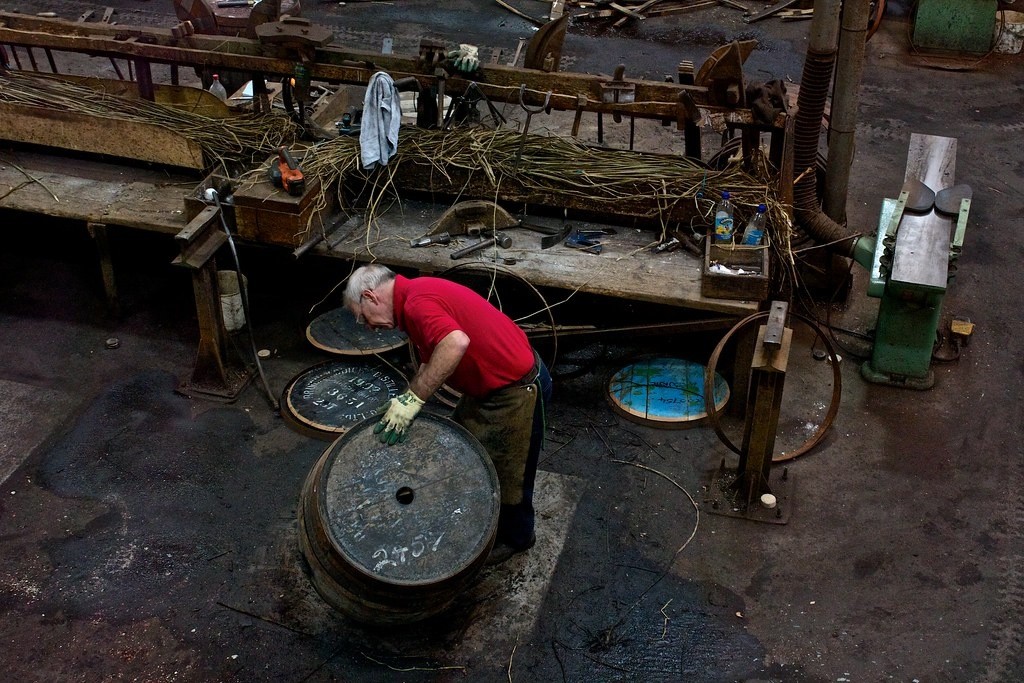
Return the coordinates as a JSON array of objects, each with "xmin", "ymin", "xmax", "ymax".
[{"xmin": 486, "ymin": 539, "xmax": 516, "ymax": 565}]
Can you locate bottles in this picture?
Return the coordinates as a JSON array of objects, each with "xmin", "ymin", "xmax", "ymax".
[
  {"xmin": 715, "ymin": 192, "xmax": 734, "ymax": 244},
  {"xmin": 739, "ymin": 205, "xmax": 767, "ymax": 245},
  {"xmin": 209, "ymin": 74, "xmax": 227, "ymax": 104}
]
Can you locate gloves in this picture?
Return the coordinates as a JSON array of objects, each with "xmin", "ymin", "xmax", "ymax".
[
  {"xmin": 447, "ymin": 44, "xmax": 479, "ymax": 72},
  {"xmin": 373, "ymin": 388, "xmax": 425, "ymax": 445}
]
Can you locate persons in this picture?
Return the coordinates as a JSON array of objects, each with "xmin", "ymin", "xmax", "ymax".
[{"xmin": 343, "ymin": 264, "xmax": 553, "ymax": 566}]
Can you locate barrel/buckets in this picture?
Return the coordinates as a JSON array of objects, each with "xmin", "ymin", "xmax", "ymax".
[{"xmin": 296, "ymin": 408, "xmax": 504, "ymax": 637}]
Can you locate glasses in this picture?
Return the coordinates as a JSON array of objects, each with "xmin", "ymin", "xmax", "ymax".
[{"xmin": 353, "ymin": 293, "xmax": 368, "ymax": 324}]
